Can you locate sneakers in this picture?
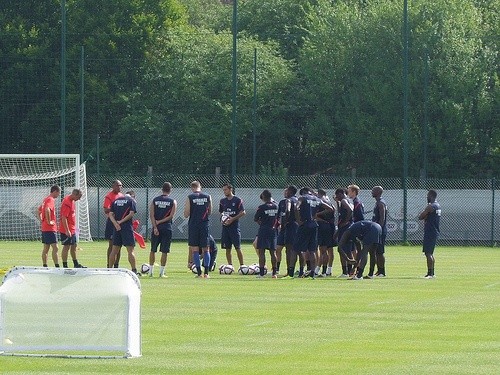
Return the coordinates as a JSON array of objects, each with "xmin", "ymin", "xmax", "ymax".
[
  {"xmin": 318, "ymin": 274, "xmax": 325, "ymax": 278},
  {"xmin": 211, "ymin": 261, "xmax": 216, "ymax": 271},
  {"xmin": 159, "ymin": 275, "xmax": 169, "ymax": 279},
  {"xmin": 256, "ymin": 274, "xmax": 266, "ymax": 278},
  {"xmin": 197, "ymin": 275, "xmax": 203, "ymax": 278},
  {"xmin": 281, "ymin": 275, "xmax": 294, "ymax": 280},
  {"xmin": 375, "ymin": 274, "xmax": 385, "ymax": 277},
  {"xmin": 424, "ymin": 275, "xmax": 434, "ymax": 279},
  {"xmin": 338, "ymin": 274, "xmax": 349, "ymax": 279},
  {"xmin": 276, "ymin": 271, "xmax": 279, "ymax": 274},
  {"xmin": 204, "ymin": 274, "xmax": 209, "ymax": 279},
  {"xmin": 298, "ymin": 275, "xmax": 303, "ymax": 278},
  {"xmin": 148, "ymin": 273, "xmax": 153, "ymax": 277},
  {"xmin": 352, "ymin": 276, "xmax": 363, "ymax": 280},
  {"xmin": 363, "ymin": 275, "xmax": 374, "ymax": 279},
  {"xmin": 272, "ymin": 275, "xmax": 277, "ymax": 278},
  {"xmin": 74, "ymin": 264, "xmax": 87, "ymax": 268}
]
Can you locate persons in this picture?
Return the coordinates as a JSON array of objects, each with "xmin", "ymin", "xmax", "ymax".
[
  {"xmin": 59, "ymin": 189, "xmax": 87, "ymax": 268},
  {"xmin": 148, "ymin": 182, "xmax": 177, "ymax": 277},
  {"xmin": 314, "ymin": 189, "xmax": 337, "ymax": 278},
  {"xmin": 184, "ymin": 181, "xmax": 212, "ymax": 279},
  {"xmin": 333, "ymin": 187, "xmax": 354, "ymax": 278},
  {"xmin": 103, "ymin": 180, "xmax": 125, "ymax": 268},
  {"xmin": 37, "ymin": 185, "xmax": 60, "ymax": 267},
  {"xmin": 253, "ymin": 186, "xmax": 323, "ymax": 276},
  {"xmin": 280, "ymin": 188, "xmax": 334, "ymax": 279},
  {"xmin": 254, "ymin": 190, "xmax": 279, "ymax": 279},
  {"xmin": 219, "ymin": 183, "xmax": 245, "ymax": 267},
  {"xmin": 418, "ymin": 190, "xmax": 441, "ymax": 279},
  {"xmin": 109, "ymin": 191, "xmax": 137, "ymax": 274},
  {"xmin": 338, "ymin": 221, "xmax": 383, "ymax": 280},
  {"xmin": 371, "ymin": 186, "xmax": 388, "ymax": 278},
  {"xmin": 284, "ymin": 185, "xmax": 304, "ymax": 278},
  {"xmin": 188, "ymin": 233, "xmax": 218, "ymax": 272},
  {"xmin": 348, "ymin": 185, "xmax": 365, "ymax": 278}
]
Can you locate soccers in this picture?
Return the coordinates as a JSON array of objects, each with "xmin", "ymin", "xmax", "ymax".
[
  {"xmin": 219, "ymin": 265, "xmax": 224, "ymax": 274},
  {"xmin": 193, "ymin": 266, "xmax": 201, "ymax": 274},
  {"xmin": 190, "ymin": 263, "xmax": 197, "ymax": 270},
  {"xmin": 224, "ymin": 265, "xmax": 235, "ymax": 274},
  {"xmin": 220, "ymin": 215, "xmax": 230, "ymax": 224},
  {"xmin": 141, "ymin": 264, "xmax": 149, "ymax": 273},
  {"xmin": 238, "ymin": 264, "xmax": 268, "ymax": 275}
]
